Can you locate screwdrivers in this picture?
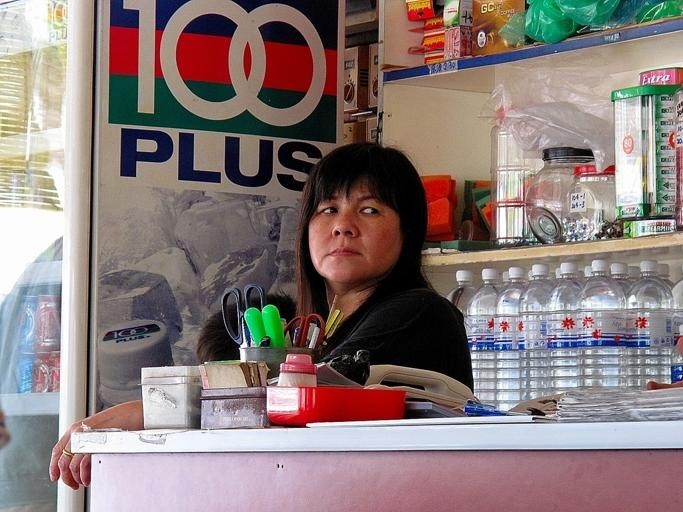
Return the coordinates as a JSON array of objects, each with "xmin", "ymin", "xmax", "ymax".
[
  {"xmin": 322, "ymin": 310, "xmax": 345, "ymax": 347},
  {"xmin": 463, "ymin": 402, "xmax": 528, "ymax": 415}
]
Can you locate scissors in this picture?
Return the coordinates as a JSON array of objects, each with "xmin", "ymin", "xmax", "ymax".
[
  {"xmin": 281, "ymin": 314, "xmax": 326, "ymax": 349},
  {"xmin": 221, "ymin": 284, "xmax": 266, "ymax": 348}
]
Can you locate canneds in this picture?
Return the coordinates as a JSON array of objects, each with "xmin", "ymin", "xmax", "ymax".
[{"xmin": 16, "ymin": 293, "xmax": 60, "ymax": 393}]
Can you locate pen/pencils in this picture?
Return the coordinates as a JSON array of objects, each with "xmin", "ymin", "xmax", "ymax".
[{"xmin": 308, "ymin": 327, "xmax": 321, "ymax": 348}]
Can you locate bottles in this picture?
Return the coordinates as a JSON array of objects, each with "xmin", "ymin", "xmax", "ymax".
[
  {"xmin": 489, "ymin": 105, "xmax": 624, "ymax": 247},
  {"xmin": 275, "ymin": 353, "xmax": 318, "ymax": 388},
  {"xmin": 0, "ymin": 0, "xmax": 77, "ymax": 210},
  {"xmin": 443, "ymin": 259, "xmax": 683, "ymax": 414},
  {"xmin": 95, "ymin": 318, "xmax": 178, "ymax": 413}
]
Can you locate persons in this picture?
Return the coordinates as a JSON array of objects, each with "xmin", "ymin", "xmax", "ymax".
[
  {"xmin": 46, "ymin": 142, "xmax": 474, "ymax": 491},
  {"xmin": 196, "ymin": 292, "xmax": 293, "ymax": 361}
]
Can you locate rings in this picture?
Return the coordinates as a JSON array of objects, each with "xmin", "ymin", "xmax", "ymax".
[{"xmin": 61, "ymin": 448, "xmax": 74, "ymax": 458}]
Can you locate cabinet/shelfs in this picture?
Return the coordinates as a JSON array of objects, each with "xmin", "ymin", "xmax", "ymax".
[{"xmin": 374, "ymin": 1, "xmax": 683, "ymax": 287}]
